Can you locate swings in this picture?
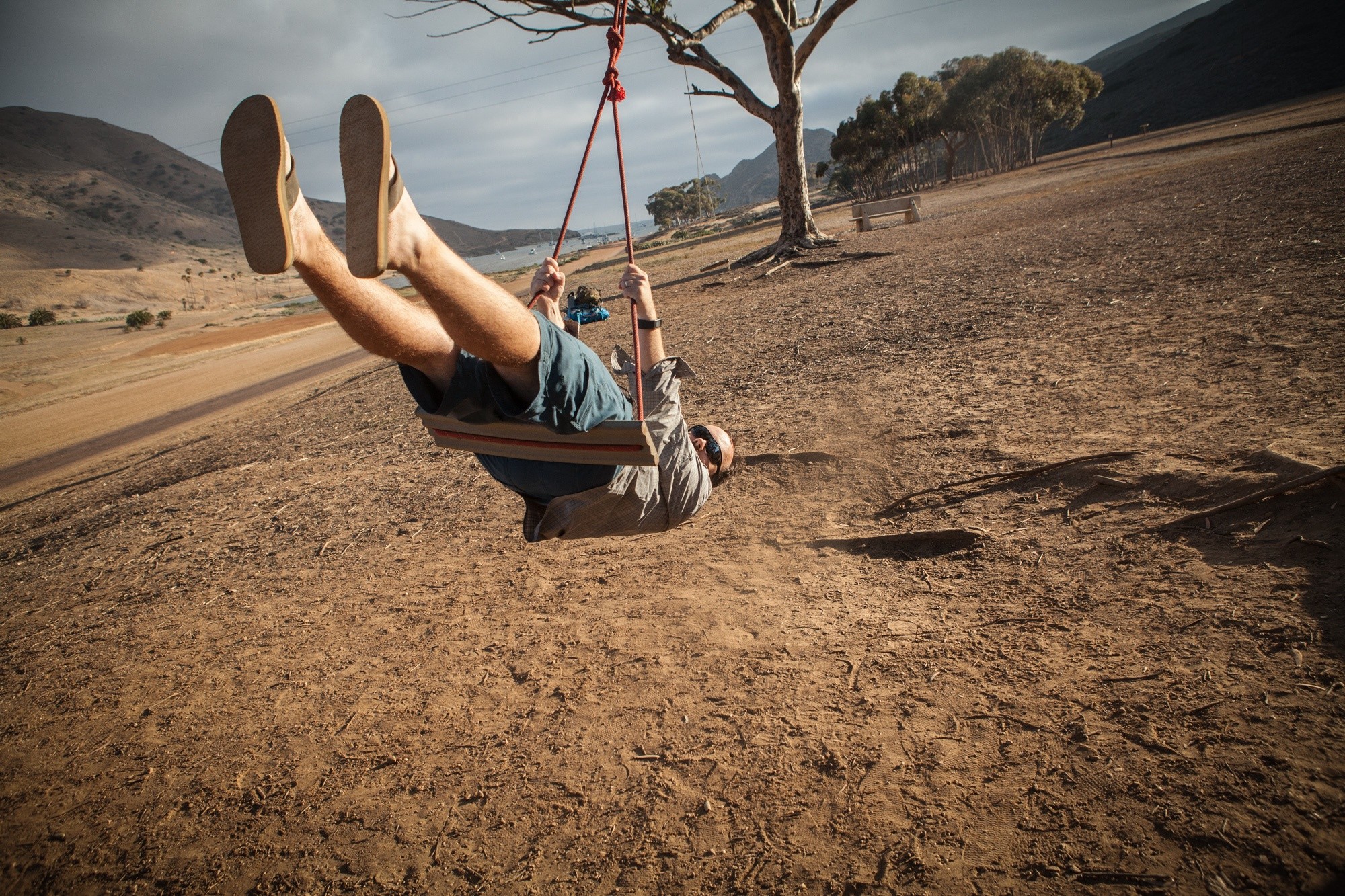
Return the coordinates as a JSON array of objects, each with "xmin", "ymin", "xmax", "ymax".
[{"xmin": 413, "ymin": 2, "xmax": 663, "ymax": 467}]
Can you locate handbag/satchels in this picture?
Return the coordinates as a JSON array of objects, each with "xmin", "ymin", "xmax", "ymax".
[{"xmin": 559, "ymin": 285, "xmax": 609, "ymax": 325}]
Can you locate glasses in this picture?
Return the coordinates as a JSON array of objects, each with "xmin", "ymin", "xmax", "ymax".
[{"xmin": 687, "ymin": 425, "xmax": 722, "ymax": 480}]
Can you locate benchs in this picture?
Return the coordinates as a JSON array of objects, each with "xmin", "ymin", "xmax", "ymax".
[{"xmin": 848, "ymin": 195, "xmax": 922, "ymax": 232}]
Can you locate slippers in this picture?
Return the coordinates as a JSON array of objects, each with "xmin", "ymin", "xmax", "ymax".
[
  {"xmin": 220, "ymin": 94, "xmax": 300, "ymax": 274},
  {"xmin": 339, "ymin": 94, "xmax": 404, "ymax": 278}
]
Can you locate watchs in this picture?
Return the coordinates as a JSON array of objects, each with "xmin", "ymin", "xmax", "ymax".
[{"xmin": 637, "ymin": 319, "xmax": 663, "ymax": 329}]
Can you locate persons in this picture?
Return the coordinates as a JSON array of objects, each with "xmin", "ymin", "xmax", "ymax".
[{"xmin": 218, "ymin": 93, "xmax": 736, "ymax": 544}]
